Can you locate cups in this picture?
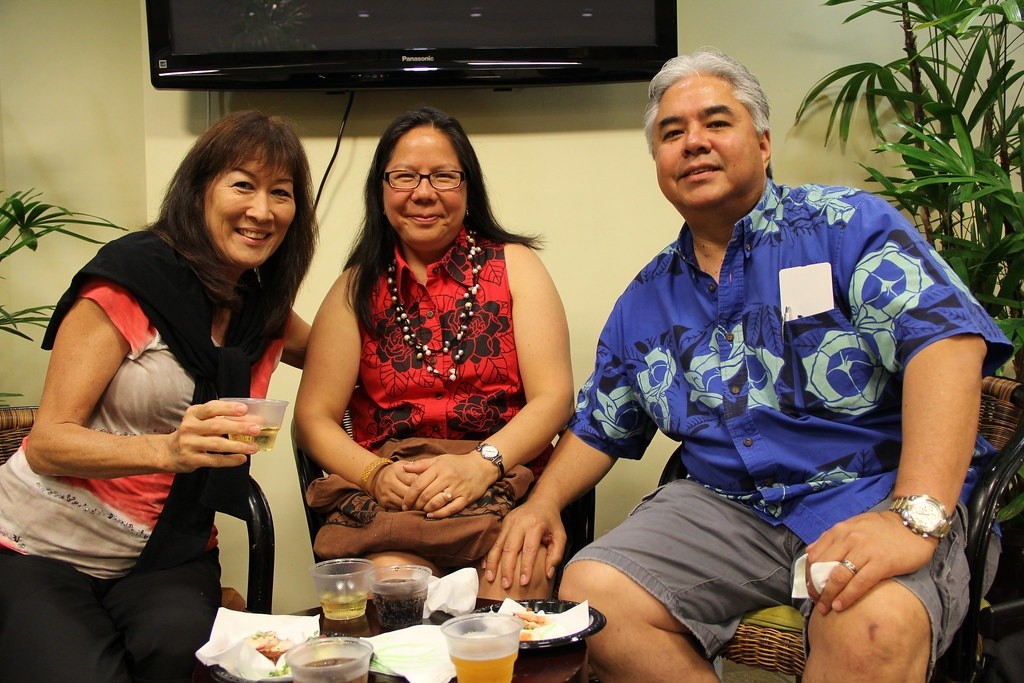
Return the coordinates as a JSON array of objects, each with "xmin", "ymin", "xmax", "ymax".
[
  {"xmin": 441, "ymin": 613, "xmax": 523, "ymax": 683},
  {"xmin": 368, "ymin": 565, "xmax": 432, "ymax": 631},
  {"xmin": 309, "ymin": 558, "xmax": 376, "ymax": 620},
  {"xmin": 219, "ymin": 399, "xmax": 289, "ymax": 452},
  {"xmin": 288, "ymin": 637, "xmax": 373, "ymax": 683}
]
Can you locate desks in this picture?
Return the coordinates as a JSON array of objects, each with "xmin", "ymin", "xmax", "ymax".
[{"xmin": 206, "ymin": 597, "xmax": 589, "ymax": 683}]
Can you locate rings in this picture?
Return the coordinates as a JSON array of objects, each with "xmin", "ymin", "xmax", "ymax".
[
  {"xmin": 840, "ymin": 559, "xmax": 858, "ymax": 575},
  {"xmin": 440, "ymin": 489, "xmax": 453, "ymax": 502}
]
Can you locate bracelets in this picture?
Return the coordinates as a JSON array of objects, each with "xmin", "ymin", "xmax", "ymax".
[
  {"xmin": 370, "ymin": 463, "xmax": 392, "ymax": 504},
  {"xmin": 360, "ymin": 457, "xmax": 393, "ymax": 498}
]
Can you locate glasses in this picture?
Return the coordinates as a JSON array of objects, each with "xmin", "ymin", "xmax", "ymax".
[{"xmin": 383, "ymin": 170, "xmax": 467, "ymax": 190}]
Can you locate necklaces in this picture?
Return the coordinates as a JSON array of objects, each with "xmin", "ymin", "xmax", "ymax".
[{"xmin": 387, "ymin": 225, "xmax": 483, "ymax": 380}]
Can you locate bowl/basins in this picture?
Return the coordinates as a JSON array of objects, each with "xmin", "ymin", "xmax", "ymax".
[
  {"xmin": 471, "ymin": 599, "xmax": 606, "ymax": 657},
  {"xmin": 369, "ymin": 671, "xmax": 407, "ymax": 683}
]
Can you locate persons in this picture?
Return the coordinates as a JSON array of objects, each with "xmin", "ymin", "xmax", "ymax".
[
  {"xmin": 483, "ymin": 50, "xmax": 1014, "ymax": 682},
  {"xmin": 293, "ymin": 108, "xmax": 574, "ymax": 604},
  {"xmin": 0, "ymin": 109, "xmax": 309, "ymax": 683}
]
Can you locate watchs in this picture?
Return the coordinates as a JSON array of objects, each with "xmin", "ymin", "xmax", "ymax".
[
  {"xmin": 888, "ymin": 494, "xmax": 952, "ymax": 539},
  {"xmin": 476, "ymin": 441, "xmax": 504, "ymax": 481}
]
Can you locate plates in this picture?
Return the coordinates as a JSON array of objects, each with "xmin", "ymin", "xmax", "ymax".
[{"xmin": 210, "ymin": 664, "xmax": 293, "ymax": 683}]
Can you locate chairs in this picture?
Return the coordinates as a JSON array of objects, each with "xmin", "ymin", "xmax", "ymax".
[
  {"xmin": 290, "ymin": 419, "xmax": 597, "ymax": 609},
  {"xmin": 0, "ymin": 406, "xmax": 276, "ymax": 683},
  {"xmin": 657, "ymin": 373, "xmax": 1024, "ymax": 683}
]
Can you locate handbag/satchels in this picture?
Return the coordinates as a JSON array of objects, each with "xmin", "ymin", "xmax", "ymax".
[{"xmin": 306, "ymin": 437, "xmax": 534, "ymax": 567}]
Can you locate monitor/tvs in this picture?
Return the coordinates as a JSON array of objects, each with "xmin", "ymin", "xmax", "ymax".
[{"xmin": 147, "ymin": 0, "xmax": 677, "ymax": 94}]
